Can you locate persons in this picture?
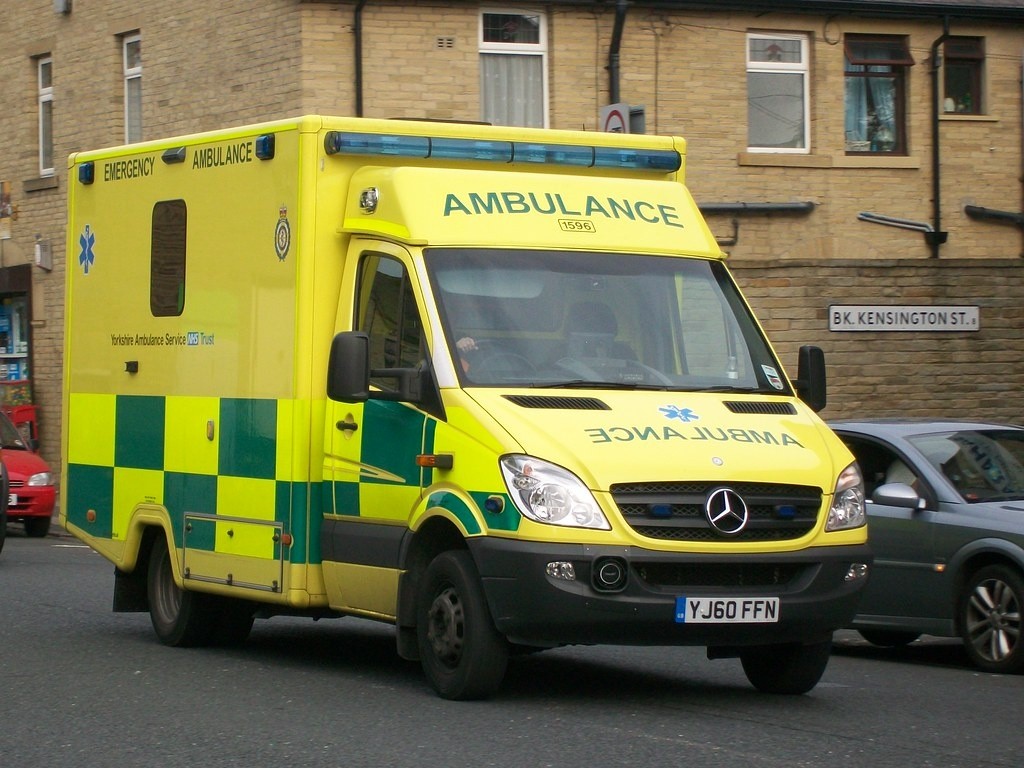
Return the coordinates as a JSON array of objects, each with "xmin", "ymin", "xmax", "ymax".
[{"xmin": 414, "ymin": 296, "xmax": 522, "ymax": 376}]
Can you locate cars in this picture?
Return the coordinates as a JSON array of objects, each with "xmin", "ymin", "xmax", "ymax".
[
  {"xmin": 0, "ymin": 409, "xmax": 57, "ymax": 536},
  {"xmin": 827, "ymin": 422, "xmax": 1024, "ymax": 673}
]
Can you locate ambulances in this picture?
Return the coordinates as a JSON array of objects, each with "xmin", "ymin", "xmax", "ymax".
[{"xmin": 56, "ymin": 115, "xmax": 874, "ymax": 704}]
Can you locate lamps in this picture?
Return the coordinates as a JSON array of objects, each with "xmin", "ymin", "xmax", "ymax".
[
  {"xmin": 925, "ymin": 231, "xmax": 948, "ymax": 247},
  {"xmin": 34, "ymin": 237, "xmax": 52, "ymax": 271}
]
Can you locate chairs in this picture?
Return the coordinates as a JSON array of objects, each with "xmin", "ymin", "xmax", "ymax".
[
  {"xmin": 870, "ymin": 452, "xmax": 966, "ymax": 510},
  {"xmin": 419, "ymin": 300, "xmax": 642, "ymax": 390}
]
